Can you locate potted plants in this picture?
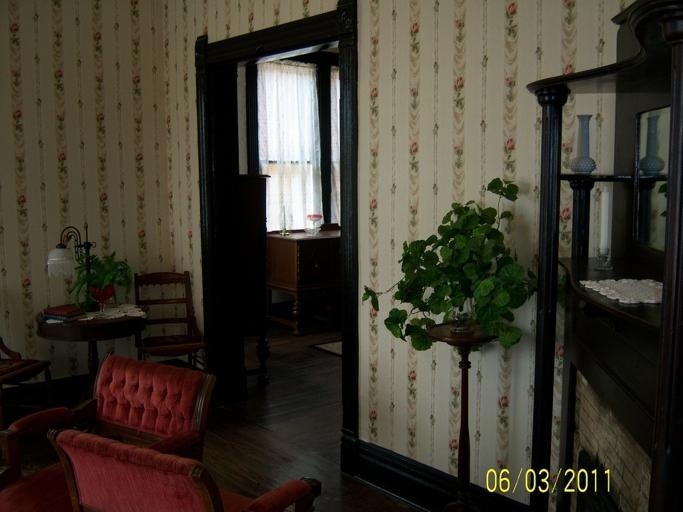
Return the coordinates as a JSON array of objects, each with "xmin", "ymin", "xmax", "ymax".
[
  {"xmin": 363, "ymin": 178, "xmax": 536, "ymax": 352},
  {"xmin": 67, "ymin": 244, "xmax": 133, "ymax": 312}
]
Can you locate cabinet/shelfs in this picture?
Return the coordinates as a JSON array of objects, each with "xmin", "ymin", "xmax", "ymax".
[{"xmin": 523, "ymin": 0, "xmax": 683, "ymax": 422}]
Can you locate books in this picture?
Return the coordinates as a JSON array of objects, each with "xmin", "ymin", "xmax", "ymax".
[
  {"xmin": 45, "ymin": 315, "xmax": 81, "ymax": 322},
  {"xmin": 45, "ymin": 304, "xmax": 80, "ymax": 316}
]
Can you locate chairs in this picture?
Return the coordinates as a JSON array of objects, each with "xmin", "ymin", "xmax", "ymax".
[
  {"xmin": 0, "ymin": 328, "xmax": 53, "ymax": 410},
  {"xmin": 134, "ymin": 271, "xmax": 203, "ymax": 367}
]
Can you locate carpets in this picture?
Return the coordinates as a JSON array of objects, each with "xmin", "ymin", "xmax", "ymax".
[{"xmin": 308, "ymin": 339, "xmax": 341, "ymax": 359}]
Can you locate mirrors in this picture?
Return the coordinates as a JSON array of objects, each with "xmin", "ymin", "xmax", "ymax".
[{"xmin": 630, "ymin": 101, "xmax": 670, "ymax": 257}]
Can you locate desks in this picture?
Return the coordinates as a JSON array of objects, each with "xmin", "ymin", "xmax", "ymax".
[
  {"xmin": 35, "ymin": 305, "xmax": 145, "ymax": 401},
  {"xmin": 266, "ymin": 230, "xmax": 341, "ymax": 337}
]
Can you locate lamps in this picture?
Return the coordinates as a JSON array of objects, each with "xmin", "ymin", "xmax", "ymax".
[{"xmin": 44, "ymin": 221, "xmax": 99, "ymax": 317}]
